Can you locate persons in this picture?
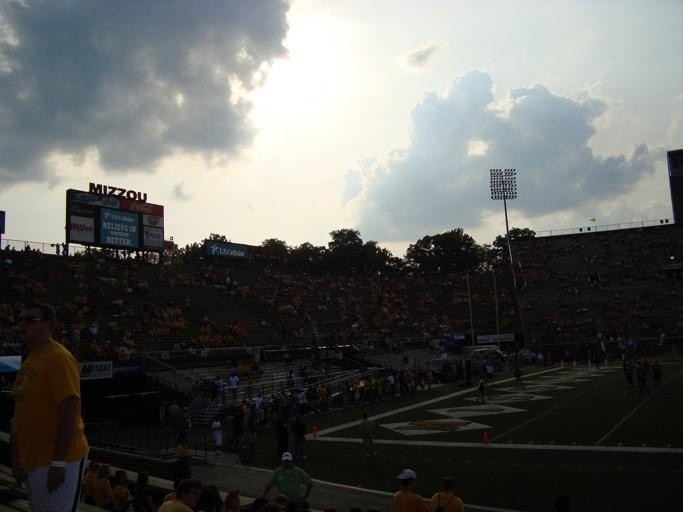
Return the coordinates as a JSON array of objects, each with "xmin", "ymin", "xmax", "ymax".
[{"xmin": 0, "ymin": 224, "xmax": 680, "ymax": 511}]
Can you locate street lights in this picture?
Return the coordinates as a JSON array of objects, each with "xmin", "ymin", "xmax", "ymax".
[{"xmin": 490, "ymin": 169, "xmax": 528, "ymax": 347}]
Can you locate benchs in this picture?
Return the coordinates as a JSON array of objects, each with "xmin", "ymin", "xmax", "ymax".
[{"xmin": 0, "ymin": 247, "xmax": 449, "ymax": 512}]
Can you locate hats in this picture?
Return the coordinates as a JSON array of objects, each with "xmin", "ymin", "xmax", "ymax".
[
  {"xmin": 396, "ymin": 469, "xmax": 417, "ymax": 480},
  {"xmin": 281, "ymin": 452, "xmax": 292, "ymax": 461}
]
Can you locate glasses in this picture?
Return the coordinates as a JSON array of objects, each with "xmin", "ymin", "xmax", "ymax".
[{"xmin": 17, "ymin": 316, "xmax": 42, "ymax": 323}]
